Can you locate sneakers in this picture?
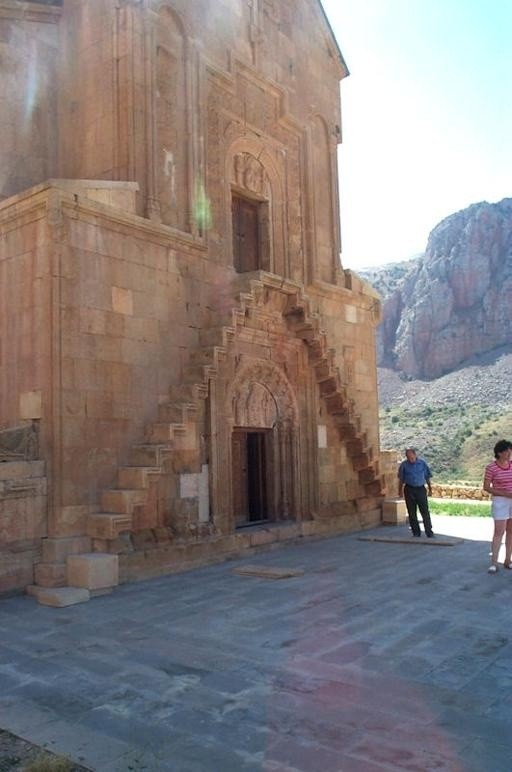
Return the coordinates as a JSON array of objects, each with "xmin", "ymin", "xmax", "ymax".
[
  {"xmin": 413, "ymin": 533, "xmax": 434, "ymax": 539},
  {"xmin": 487, "ymin": 560, "xmax": 512, "ymax": 575}
]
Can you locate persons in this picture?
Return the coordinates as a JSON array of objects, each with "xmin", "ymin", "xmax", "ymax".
[
  {"xmin": 483, "ymin": 439, "xmax": 512, "ymax": 573},
  {"xmin": 397, "ymin": 448, "xmax": 433, "ymax": 538}
]
